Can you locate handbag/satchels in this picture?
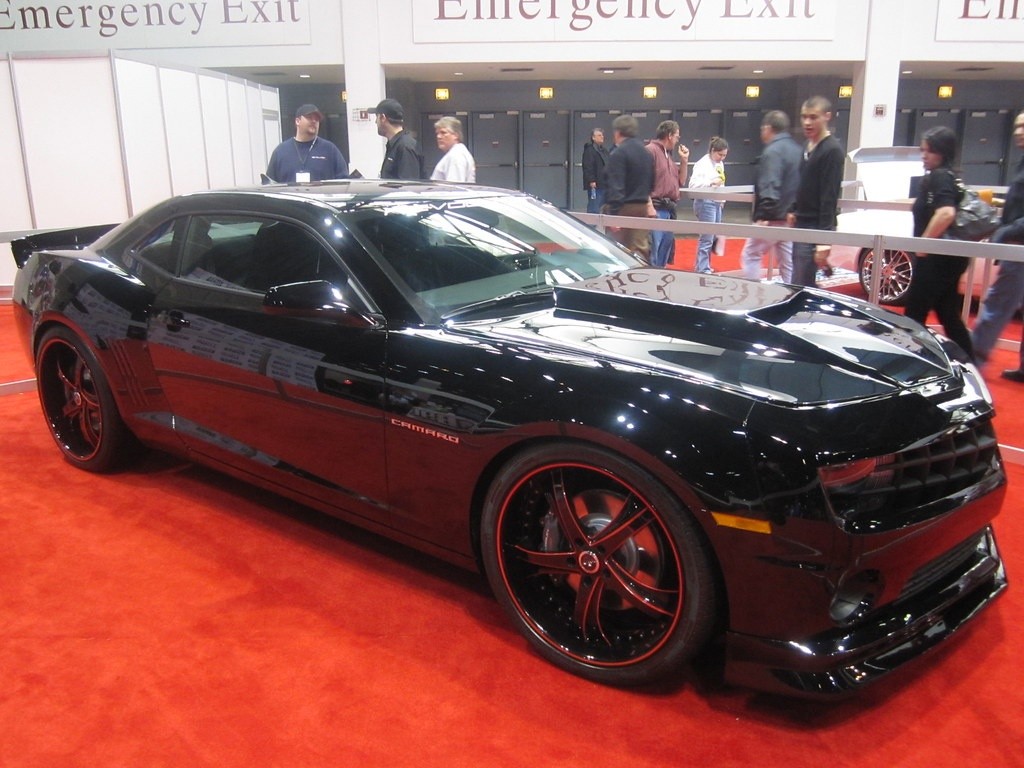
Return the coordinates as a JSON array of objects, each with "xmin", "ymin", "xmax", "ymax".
[
  {"xmin": 929, "ymin": 168, "xmax": 1002, "ymax": 243},
  {"xmin": 712, "ymin": 235, "xmax": 726, "ymax": 256}
]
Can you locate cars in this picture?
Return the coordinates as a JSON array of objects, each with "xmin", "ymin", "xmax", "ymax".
[
  {"xmin": 11, "ymin": 179, "xmax": 1007, "ymax": 702},
  {"xmin": 826, "ymin": 145, "xmax": 1024, "ymax": 313}
]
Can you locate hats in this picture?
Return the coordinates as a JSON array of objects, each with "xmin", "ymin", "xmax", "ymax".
[
  {"xmin": 295, "ymin": 104, "xmax": 323, "ymax": 121},
  {"xmin": 366, "ymin": 99, "xmax": 403, "ymax": 119}
]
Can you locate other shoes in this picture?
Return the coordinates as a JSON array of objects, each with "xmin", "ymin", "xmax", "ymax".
[
  {"xmin": 703, "ymin": 267, "xmax": 714, "ymax": 275},
  {"xmin": 1002, "ymin": 368, "xmax": 1024, "ymax": 382}
]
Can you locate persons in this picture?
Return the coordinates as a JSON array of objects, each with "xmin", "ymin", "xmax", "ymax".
[
  {"xmin": 971, "ymin": 112, "xmax": 1024, "ymax": 383},
  {"xmin": 739, "ymin": 110, "xmax": 802, "ymax": 283},
  {"xmin": 787, "ymin": 95, "xmax": 842, "ymax": 288},
  {"xmin": 430, "ymin": 116, "xmax": 475, "ymax": 184},
  {"xmin": 688, "ymin": 136, "xmax": 728, "ymax": 274},
  {"xmin": 645, "ymin": 120, "xmax": 690, "ymax": 269},
  {"xmin": 583, "ymin": 128, "xmax": 609, "ymax": 227},
  {"xmin": 265, "ymin": 104, "xmax": 348, "ymax": 181},
  {"xmin": 368, "ymin": 98, "xmax": 428, "ymax": 181},
  {"xmin": 902, "ymin": 127, "xmax": 973, "ymax": 351},
  {"xmin": 603, "ymin": 114, "xmax": 655, "ymax": 255}
]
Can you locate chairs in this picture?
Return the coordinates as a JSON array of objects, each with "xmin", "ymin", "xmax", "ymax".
[
  {"xmin": 252, "ymin": 223, "xmax": 323, "ymax": 290},
  {"xmin": 376, "ymin": 217, "xmax": 445, "ymax": 292}
]
